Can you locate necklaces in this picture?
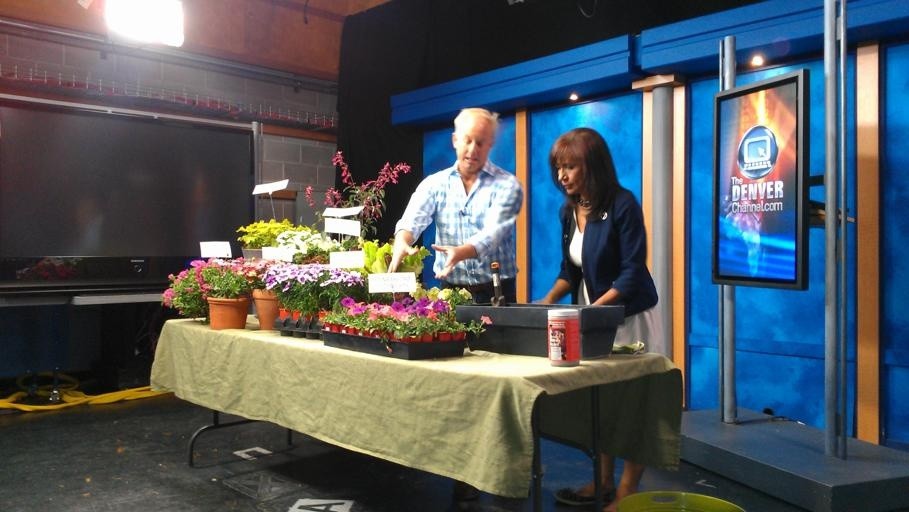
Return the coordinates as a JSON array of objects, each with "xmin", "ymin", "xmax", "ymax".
[{"xmin": 579, "ymin": 199, "xmax": 592, "ymax": 207}]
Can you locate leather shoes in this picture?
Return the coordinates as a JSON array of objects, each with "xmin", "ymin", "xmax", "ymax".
[
  {"xmin": 451, "ymin": 480, "xmax": 478, "ymax": 502},
  {"xmin": 554, "ymin": 485, "xmax": 616, "ymax": 506}
]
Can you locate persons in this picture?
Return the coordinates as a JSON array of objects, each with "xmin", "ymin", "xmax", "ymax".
[
  {"xmin": 387, "ymin": 106, "xmax": 523, "ymax": 501},
  {"xmin": 537, "ymin": 127, "xmax": 660, "ymax": 511}
]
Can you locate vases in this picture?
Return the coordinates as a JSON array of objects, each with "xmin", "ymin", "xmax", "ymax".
[
  {"xmin": 251, "ymin": 288, "xmax": 281, "ymax": 330},
  {"xmin": 277, "ymin": 309, "xmax": 330, "ymax": 329},
  {"xmin": 205, "ymin": 297, "xmax": 248, "ymax": 328},
  {"xmin": 319, "ymin": 323, "xmax": 469, "ymax": 343},
  {"xmin": 240, "ymin": 246, "xmax": 263, "ymax": 260}
]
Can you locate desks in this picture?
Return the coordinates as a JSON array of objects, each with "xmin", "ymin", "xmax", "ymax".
[{"xmin": 151, "ymin": 308, "xmax": 682, "ymax": 511}]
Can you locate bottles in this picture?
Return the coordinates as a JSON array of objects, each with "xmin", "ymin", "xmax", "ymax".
[
  {"xmin": 30, "ymin": 360, "xmax": 41, "ymax": 397},
  {"xmin": 49, "ymin": 367, "xmax": 62, "ymax": 403}
]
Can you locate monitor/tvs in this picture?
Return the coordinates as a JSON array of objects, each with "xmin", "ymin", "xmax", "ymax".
[
  {"xmin": 711, "ymin": 69, "xmax": 810, "ymax": 292},
  {"xmin": 0, "ymin": 92, "xmax": 264, "ymax": 298}
]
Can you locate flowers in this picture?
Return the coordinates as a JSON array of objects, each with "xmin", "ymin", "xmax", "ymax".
[
  {"xmin": 163, "ymin": 257, "xmax": 251, "ymax": 316},
  {"xmin": 323, "ymin": 296, "xmax": 488, "ymax": 334},
  {"xmin": 275, "ymin": 229, "xmax": 338, "ymax": 250},
  {"xmin": 263, "ymin": 263, "xmax": 366, "ymax": 311},
  {"xmin": 304, "ymin": 150, "xmax": 409, "ymax": 251},
  {"xmin": 236, "ymin": 220, "xmax": 308, "ymax": 247},
  {"xmin": 245, "ymin": 261, "xmax": 282, "ymax": 292}
]
